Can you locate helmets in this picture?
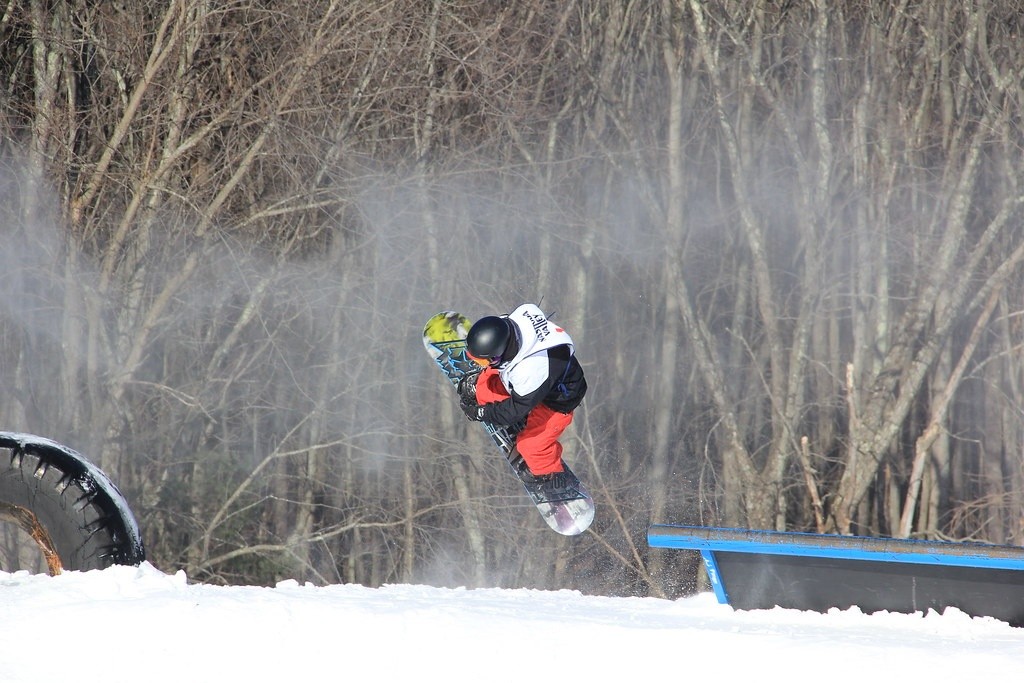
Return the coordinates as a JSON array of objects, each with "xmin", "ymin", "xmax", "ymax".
[{"xmin": 466, "ymin": 316, "xmax": 509, "ymax": 358}]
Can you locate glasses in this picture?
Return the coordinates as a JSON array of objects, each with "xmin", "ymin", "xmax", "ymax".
[{"xmin": 465, "ymin": 350, "xmax": 490, "ymax": 368}]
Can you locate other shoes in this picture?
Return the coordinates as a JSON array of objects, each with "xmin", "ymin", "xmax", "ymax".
[{"xmin": 521, "ymin": 465, "xmax": 552, "ymax": 482}]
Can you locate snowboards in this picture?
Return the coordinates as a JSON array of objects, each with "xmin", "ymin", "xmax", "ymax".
[{"xmin": 422, "ymin": 310, "xmax": 594, "ymax": 536}]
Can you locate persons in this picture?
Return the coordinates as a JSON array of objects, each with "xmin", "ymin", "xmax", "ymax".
[{"xmin": 458, "ymin": 303, "xmax": 587, "ymax": 484}]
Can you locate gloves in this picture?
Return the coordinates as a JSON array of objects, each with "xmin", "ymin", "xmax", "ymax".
[{"xmin": 459, "ymin": 397, "xmax": 484, "ymax": 423}]
